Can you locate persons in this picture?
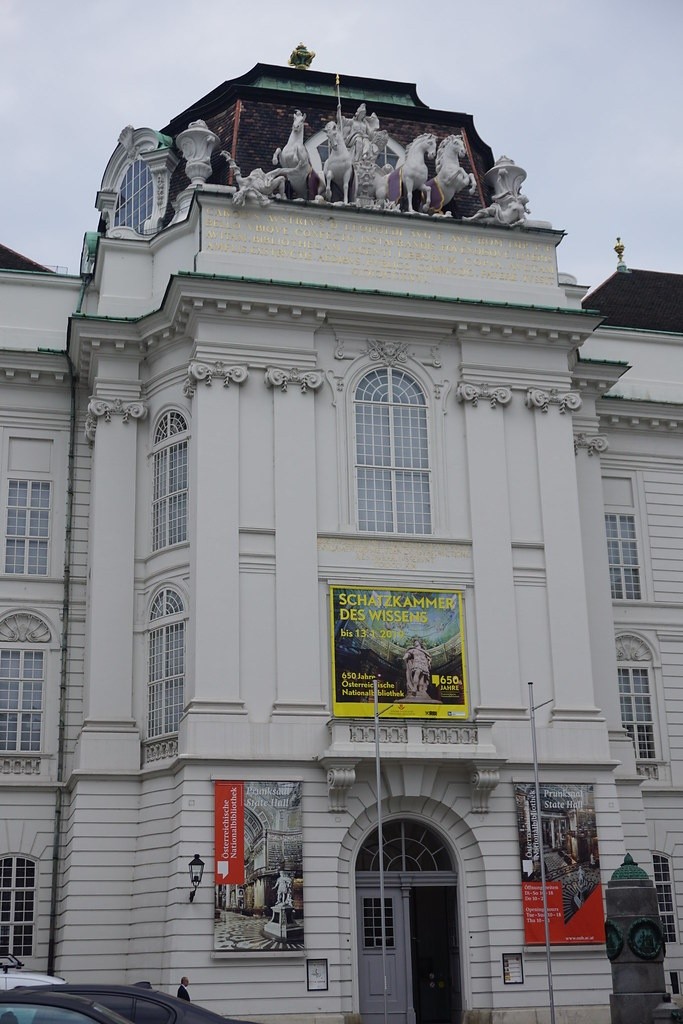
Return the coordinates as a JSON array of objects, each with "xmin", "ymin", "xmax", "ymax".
[
  {"xmin": 271, "ymin": 871, "xmax": 291, "ymax": 904},
  {"xmin": 177, "ymin": 977, "xmax": 191, "ymax": 1002},
  {"xmin": 403, "ymin": 639, "xmax": 432, "ymax": 693}
]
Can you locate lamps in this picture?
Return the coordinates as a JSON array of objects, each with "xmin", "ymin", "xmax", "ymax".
[{"xmin": 188, "ymin": 854, "xmax": 205, "ymax": 902}]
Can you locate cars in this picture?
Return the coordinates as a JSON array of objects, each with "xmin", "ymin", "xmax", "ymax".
[
  {"xmin": 7, "ymin": 981, "xmax": 259, "ymax": 1024},
  {"xmin": 0, "ymin": 991, "xmax": 134, "ymax": 1024},
  {"xmin": 1, "ymin": 970, "xmax": 71, "ymax": 994}
]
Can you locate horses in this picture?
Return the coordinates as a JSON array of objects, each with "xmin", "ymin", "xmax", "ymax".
[
  {"xmin": 418, "ymin": 133, "xmax": 477, "ymax": 217},
  {"xmin": 272, "ymin": 110, "xmax": 326, "ymax": 202},
  {"xmin": 375, "ymin": 133, "xmax": 438, "ymax": 216},
  {"xmin": 320, "ymin": 121, "xmax": 354, "ymax": 206}
]
